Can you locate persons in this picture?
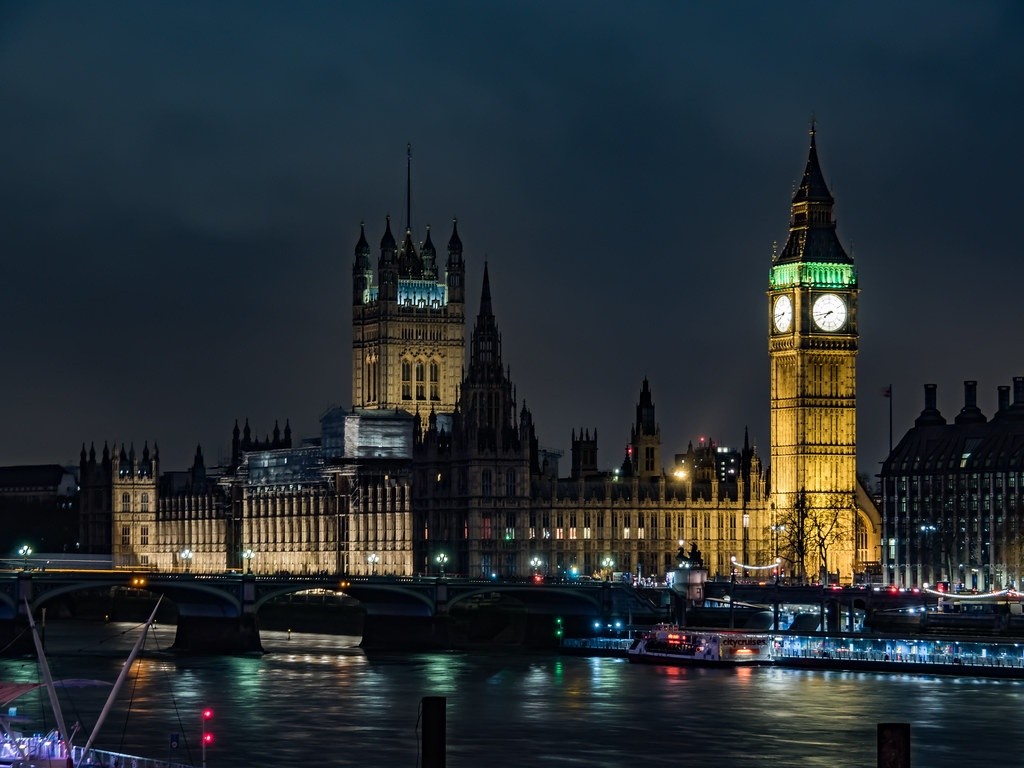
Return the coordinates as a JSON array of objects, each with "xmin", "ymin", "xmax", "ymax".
[
  {"xmin": 662, "ymin": 579, "xmax": 667, "ymax": 585},
  {"xmin": 418, "ymin": 573, "xmax": 422, "ymax": 582},
  {"xmin": 592, "ymin": 569, "xmax": 601, "ymax": 580},
  {"xmin": 642, "ymin": 576, "xmax": 651, "ymax": 586}
]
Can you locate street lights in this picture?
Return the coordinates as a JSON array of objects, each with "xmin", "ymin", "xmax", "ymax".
[
  {"xmin": 775, "ymin": 557, "xmax": 782, "ymax": 583},
  {"xmin": 731, "ymin": 556, "xmax": 736, "ymax": 582},
  {"xmin": 437, "ymin": 552, "xmax": 449, "ymax": 578},
  {"xmin": 243, "ymin": 549, "xmax": 256, "ymax": 574},
  {"xmin": 368, "ymin": 553, "xmax": 381, "ymax": 576},
  {"xmin": 531, "ymin": 557, "xmax": 541, "ymax": 576},
  {"xmin": 180, "ymin": 549, "xmax": 193, "ymax": 572},
  {"xmin": 19, "ymin": 545, "xmax": 31, "ymax": 571},
  {"xmin": 772, "ymin": 522, "xmax": 785, "ymax": 557},
  {"xmin": 603, "ymin": 557, "xmax": 614, "ymax": 581}
]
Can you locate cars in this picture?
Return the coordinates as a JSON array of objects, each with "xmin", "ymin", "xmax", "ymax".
[
  {"xmin": 880, "ymin": 586, "xmax": 899, "ymax": 594},
  {"xmin": 905, "ymin": 587, "xmax": 919, "ymax": 594},
  {"xmin": 825, "ymin": 584, "xmax": 842, "ymax": 591}
]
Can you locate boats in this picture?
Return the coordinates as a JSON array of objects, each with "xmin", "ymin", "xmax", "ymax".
[{"xmin": 629, "ymin": 621, "xmax": 775, "ymax": 668}]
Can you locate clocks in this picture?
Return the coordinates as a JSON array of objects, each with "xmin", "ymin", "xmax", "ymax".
[
  {"xmin": 774, "ymin": 295, "xmax": 791, "ymax": 332},
  {"xmin": 813, "ymin": 292, "xmax": 848, "ymax": 332}
]
